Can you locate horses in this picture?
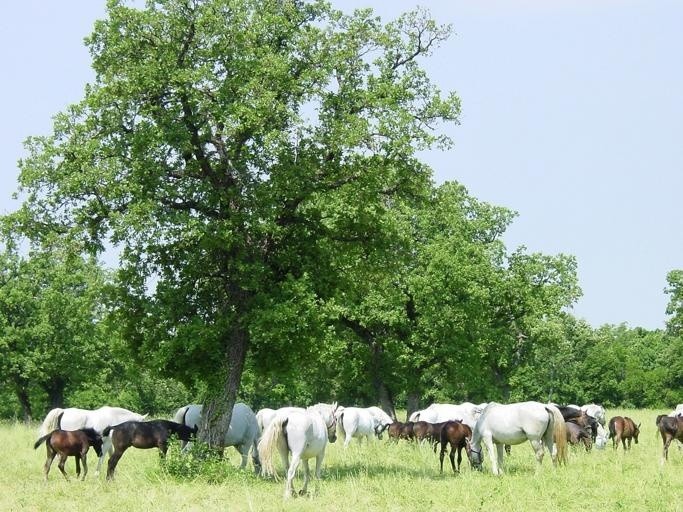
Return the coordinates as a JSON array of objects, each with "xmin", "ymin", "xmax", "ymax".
[
  {"xmin": 33, "ymin": 401, "xmax": 610, "ymax": 499},
  {"xmin": 609, "ymin": 416, "xmax": 641, "ymax": 451},
  {"xmin": 656, "ymin": 413, "xmax": 683, "ymax": 465}
]
